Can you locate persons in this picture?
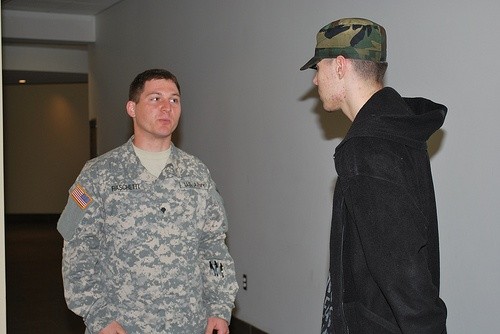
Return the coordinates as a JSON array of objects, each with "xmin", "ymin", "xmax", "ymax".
[
  {"xmin": 298, "ymin": 18, "xmax": 449, "ymax": 334},
  {"xmin": 57, "ymin": 70, "xmax": 240, "ymax": 334}
]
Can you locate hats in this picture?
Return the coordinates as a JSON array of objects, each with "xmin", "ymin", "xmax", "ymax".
[{"xmin": 299, "ymin": 17, "xmax": 386, "ymax": 71}]
